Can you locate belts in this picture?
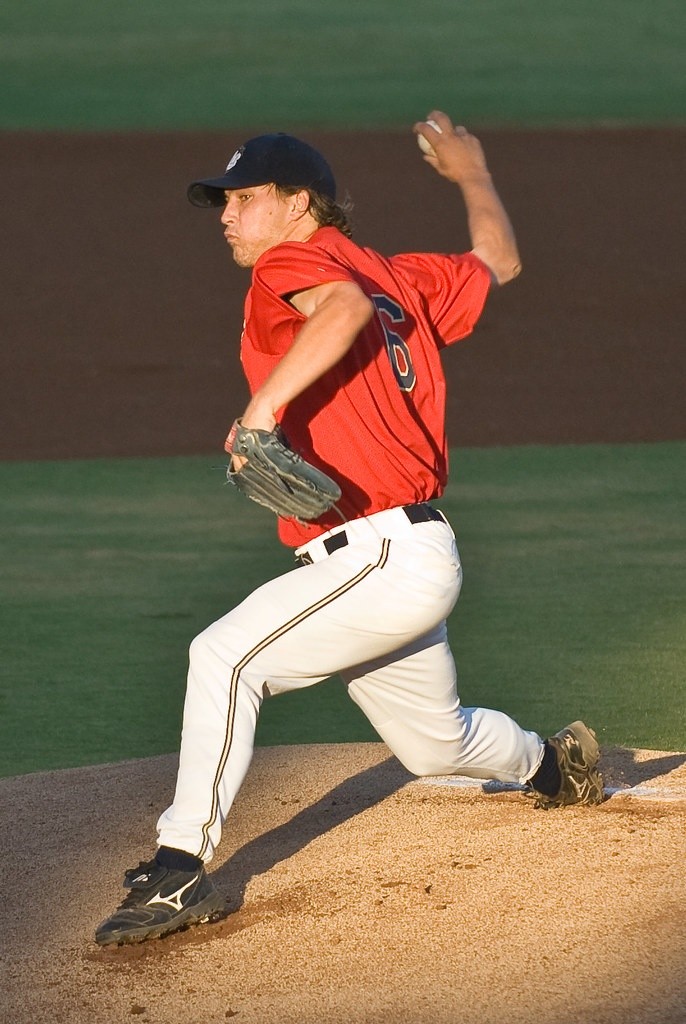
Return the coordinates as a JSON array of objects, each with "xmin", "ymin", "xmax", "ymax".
[{"xmin": 294, "ymin": 503, "xmax": 447, "ymax": 568}]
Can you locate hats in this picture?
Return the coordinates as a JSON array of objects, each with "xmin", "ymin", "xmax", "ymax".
[{"xmin": 186, "ymin": 133, "xmax": 337, "ymax": 209}]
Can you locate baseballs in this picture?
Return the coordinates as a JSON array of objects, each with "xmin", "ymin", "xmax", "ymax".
[{"xmin": 414, "ymin": 116, "xmax": 451, "ymax": 161}]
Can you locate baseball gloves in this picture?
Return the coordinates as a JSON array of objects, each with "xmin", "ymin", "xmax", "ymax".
[{"xmin": 219, "ymin": 413, "xmax": 347, "ymax": 527}]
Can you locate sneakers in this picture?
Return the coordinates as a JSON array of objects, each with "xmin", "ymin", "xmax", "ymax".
[
  {"xmin": 94, "ymin": 859, "xmax": 225, "ymax": 950},
  {"xmin": 521, "ymin": 719, "xmax": 604, "ymax": 812}
]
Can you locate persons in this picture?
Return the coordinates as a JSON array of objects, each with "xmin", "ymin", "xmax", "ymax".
[{"xmin": 95, "ymin": 110, "xmax": 603, "ymax": 943}]
